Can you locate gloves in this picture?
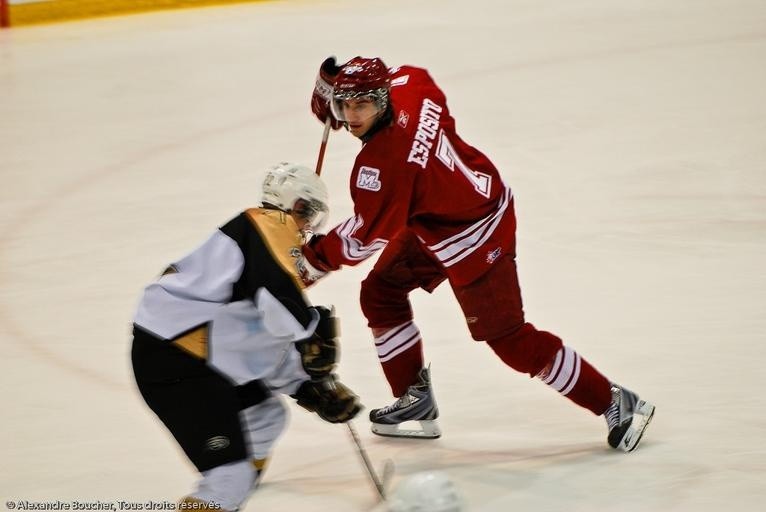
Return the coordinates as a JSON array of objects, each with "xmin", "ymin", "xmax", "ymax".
[
  {"xmin": 290, "ymin": 376, "xmax": 364, "ymax": 424},
  {"xmin": 294, "ymin": 305, "xmax": 337, "ymax": 376},
  {"xmin": 296, "ymin": 233, "xmax": 327, "ymax": 288},
  {"xmin": 310, "ymin": 57, "xmax": 348, "ymax": 130}
]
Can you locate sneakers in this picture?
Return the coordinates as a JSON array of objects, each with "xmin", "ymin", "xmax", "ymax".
[
  {"xmin": 369, "ymin": 367, "xmax": 440, "ymax": 425},
  {"xmin": 602, "ymin": 378, "xmax": 640, "ymax": 449}
]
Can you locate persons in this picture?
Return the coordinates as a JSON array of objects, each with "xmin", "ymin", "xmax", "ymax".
[
  {"xmin": 131, "ymin": 164, "xmax": 364, "ymax": 512},
  {"xmin": 296, "ymin": 56, "xmax": 640, "ymax": 450}
]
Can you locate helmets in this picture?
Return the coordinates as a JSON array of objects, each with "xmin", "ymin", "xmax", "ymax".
[
  {"xmin": 260, "ymin": 160, "xmax": 330, "ymax": 213},
  {"xmin": 334, "ymin": 57, "xmax": 392, "ymax": 104}
]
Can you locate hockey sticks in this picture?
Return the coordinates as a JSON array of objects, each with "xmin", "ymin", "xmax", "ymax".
[{"xmin": 320, "ymin": 377, "xmax": 393, "ymax": 501}]
